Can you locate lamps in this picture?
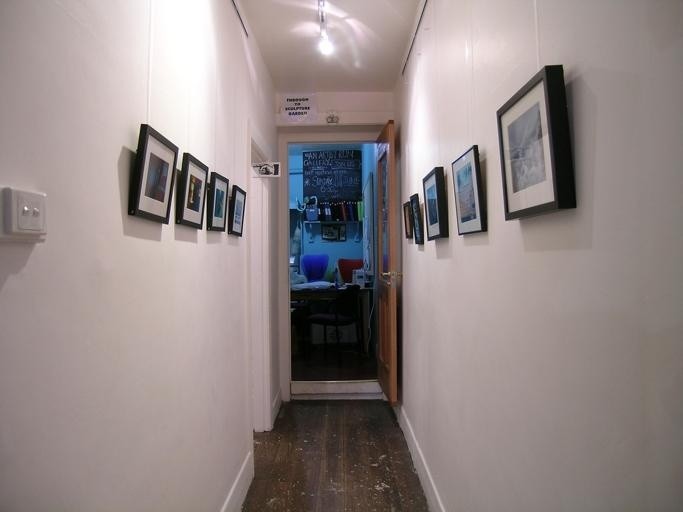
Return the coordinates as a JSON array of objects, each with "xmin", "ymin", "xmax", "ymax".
[{"xmin": 318, "ymin": 0, "xmax": 334, "ymax": 55}]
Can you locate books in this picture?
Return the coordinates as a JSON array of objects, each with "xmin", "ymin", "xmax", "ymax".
[{"xmin": 316, "ymin": 200, "xmax": 363, "ymax": 222}]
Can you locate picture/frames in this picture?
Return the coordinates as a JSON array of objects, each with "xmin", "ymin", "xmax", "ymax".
[
  {"xmin": 403, "ymin": 65, "xmax": 577, "ymax": 245},
  {"xmin": 127, "ymin": 123, "xmax": 246, "ymax": 237}
]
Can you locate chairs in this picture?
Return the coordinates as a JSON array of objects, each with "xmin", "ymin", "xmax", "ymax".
[{"xmin": 300, "ymin": 253, "xmax": 366, "ymax": 367}]
